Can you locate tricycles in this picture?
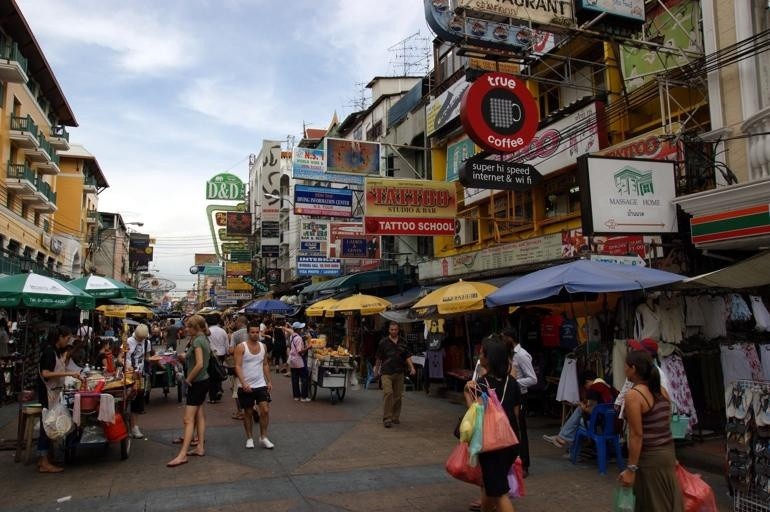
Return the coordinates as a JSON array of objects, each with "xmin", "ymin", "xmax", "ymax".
[
  {"xmin": 144, "ymin": 347, "xmax": 184, "ymax": 404},
  {"xmin": 55, "ymin": 381, "xmax": 137, "ymax": 463},
  {"xmin": 305, "ymin": 354, "xmax": 355, "ymax": 406}
]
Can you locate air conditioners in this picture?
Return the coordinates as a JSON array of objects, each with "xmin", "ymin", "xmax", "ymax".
[{"xmin": 454, "ymin": 217, "xmax": 473, "ymax": 247}]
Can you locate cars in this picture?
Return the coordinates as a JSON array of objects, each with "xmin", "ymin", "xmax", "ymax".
[{"xmin": 155, "ymin": 299, "xmax": 196, "ymax": 338}]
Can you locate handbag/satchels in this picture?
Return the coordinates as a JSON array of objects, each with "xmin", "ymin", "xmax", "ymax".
[
  {"xmin": 669, "ymin": 415, "xmax": 689, "ymax": 439},
  {"xmin": 208, "ymin": 357, "xmax": 227, "ymax": 383},
  {"xmin": 459, "ymin": 377, "xmax": 519, "ymax": 467},
  {"xmin": 445, "ymin": 441, "xmax": 525, "ymax": 496}
]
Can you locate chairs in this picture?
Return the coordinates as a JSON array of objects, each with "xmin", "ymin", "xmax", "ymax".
[
  {"xmin": 364, "ymin": 359, "xmax": 381, "ymax": 390},
  {"xmin": 572, "ymin": 403, "xmax": 624, "ymax": 475}
]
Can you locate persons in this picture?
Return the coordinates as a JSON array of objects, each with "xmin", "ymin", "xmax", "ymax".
[
  {"xmin": 161, "ymin": 313, "xmax": 260, "ymax": 422},
  {"xmin": 36, "ymin": 322, "xmax": 160, "ymax": 473},
  {"xmin": 374, "ymin": 321, "xmax": 416, "ymax": 428},
  {"xmin": 617, "ymin": 339, "xmax": 685, "ymax": 512},
  {"xmin": 463, "ymin": 329, "xmax": 537, "ymax": 512},
  {"xmin": 235, "ymin": 322, "xmax": 274, "ymax": 448},
  {"xmin": 260, "ymin": 318, "xmax": 319, "ymax": 402},
  {"xmin": 166, "ymin": 315, "xmax": 219, "ymax": 466},
  {"xmin": 543, "ymin": 370, "xmax": 624, "ymax": 460}
]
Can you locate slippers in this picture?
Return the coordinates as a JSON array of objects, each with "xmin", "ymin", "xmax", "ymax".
[
  {"xmin": 167, "ymin": 437, "xmax": 205, "ymax": 467},
  {"xmin": 725, "ymin": 384, "xmax": 770, "ymax": 501},
  {"xmin": 469, "ymin": 501, "xmax": 496, "ymax": 511},
  {"xmin": 543, "ymin": 435, "xmax": 563, "ymax": 449}
]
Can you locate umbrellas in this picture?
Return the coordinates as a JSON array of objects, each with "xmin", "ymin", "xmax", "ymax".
[
  {"xmin": 0, "ymin": 270, "xmax": 96, "ymax": 402},
  {"xmin": 305, "ymin": 297, "xmax": 339, "ymax": 317},
  {"xmin": 486, "ymin": 258, "xmax": 689, "ymax": 369},
  {"xmin": 67, "ymin": 273, "xmax": 158, "ymax": 320},
  {"xmin": 245, "ymin": 298, "xmax": 294, "ymax": 316},
  {"xmin": 407, "ymin": 279, "xmax": 520, "ymax": 372},
  {"xmin": 326, "ymin": 292, "xmax": 392, "ymax": 354}
]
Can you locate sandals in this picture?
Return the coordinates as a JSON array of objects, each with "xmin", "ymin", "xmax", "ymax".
[
  {"xmin": 231, "ymin": 413, "xmax": 244, "ymax": 420},
  {"xmin": 251, "ymin": 408, "xmax": 259, "ymax": 423}
]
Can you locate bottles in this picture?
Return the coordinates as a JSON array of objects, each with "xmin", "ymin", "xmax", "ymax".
[{"xmin": 83, "ymin": 363, "xmax": 108, "ymax": 377}]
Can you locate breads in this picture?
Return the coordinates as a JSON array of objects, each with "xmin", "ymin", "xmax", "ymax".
[{"xmin": 313, "ymin": 346, "xmax": 350, "ymax": 358}]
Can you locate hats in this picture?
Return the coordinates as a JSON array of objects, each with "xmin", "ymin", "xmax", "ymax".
[
  {"xmin": 628, "ymin": 338, "xmax": 657, "ymax": 357},
  {"xmin": 292, "ymin": 322, "xmax": 306, "ymax": 330}
]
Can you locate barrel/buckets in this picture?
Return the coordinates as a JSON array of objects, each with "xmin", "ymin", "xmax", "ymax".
[
  {"xmin": 103, "ymin": 412, "xmax": 128, "ymax": 443},
  {"xmin": 74, "ymin": 389, "xmax": 101, "ymax": 411}
]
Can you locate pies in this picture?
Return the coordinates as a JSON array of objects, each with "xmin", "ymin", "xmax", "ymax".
[{"xmin": 44, "ymin": 415, "xmax": 72, "ymax": 440}]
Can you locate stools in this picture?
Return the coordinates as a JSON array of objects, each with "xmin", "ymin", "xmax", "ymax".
[{"xmin": 15, "ymin": 407, "xmax": 42, "ymax": 466}]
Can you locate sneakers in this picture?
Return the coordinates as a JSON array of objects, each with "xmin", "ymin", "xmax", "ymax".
[
  {"xmin": 131, "ymin": 425, "xmax": 143, "ymax": 439},
  {"xmin": 258, "ymin": 437, "xmax": 274, "ymax": 448},
  {"xmin": 41, "ymin": 465, "xmax": 63, "ymax": 473},
  {"xmin": 384, "ymin": 419, "xmax": 399, "ymax": 427},
  {"xmin": 294, "ymin": 397, "xmax": 311, "ymax": 402},
  {"xmin": 245, "ymin": 438, "xmax": 254, "ymax": 448}
]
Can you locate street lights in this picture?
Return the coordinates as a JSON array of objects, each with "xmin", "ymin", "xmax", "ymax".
[
  {"xmin": 92, "ymin": 222, "xmax": 144, "ymax": 330},
  {"xmin": 133, "ymin": 269, "xmax": 161, "ymax": 289},
  {"xmin": 263, "ymin": 193, "xmax": 307, "ymax": 221}
]
